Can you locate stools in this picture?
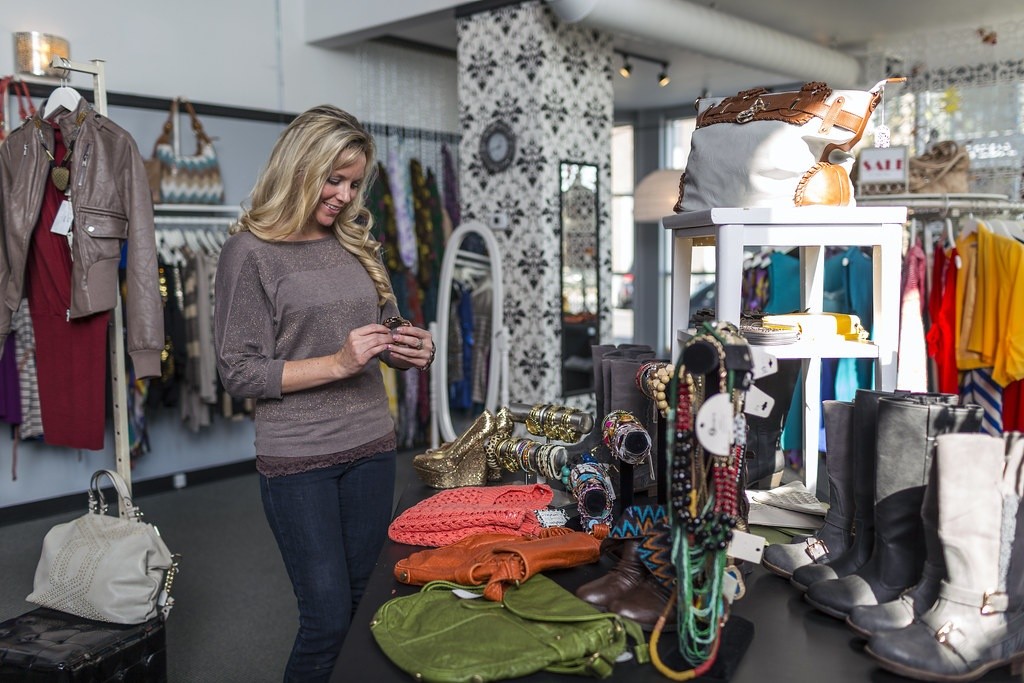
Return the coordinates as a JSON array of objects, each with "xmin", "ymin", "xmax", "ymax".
[{"xmin": 0, "ymin": 607, "xmax": 167, "ymax": 683}]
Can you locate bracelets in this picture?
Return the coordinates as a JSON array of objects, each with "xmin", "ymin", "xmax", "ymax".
[
  {"xmin": 571, "ymin": 463, "xmax": 613, "ymax": 526},
  {"xmin": 417, "ymin": 341, "xmax": 436, "ymax": 372},
  {"xmin": 518, "ymin": 441, "xmax": 567, "ymax": 480},
  {"xmin": 601, "ymin": 409, "xmax": 653, "ymax": 466},
  {"xmin": 636, "ymin": 361, "xmax": 700, "ymax": 416}
]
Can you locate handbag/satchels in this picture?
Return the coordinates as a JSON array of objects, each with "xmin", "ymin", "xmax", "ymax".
[
  {"xmin": 673, "ymin": 77, "xmax": 908, "ymax": 213},
  {"xmin": 741, "ymin": 323, "xmax": 802, "ymax": 346},
  {"xmin": 24, "ymin": 470, "xmax": 180, "ymax": 627},
  {"xmin": 763, "ymin": 309, "xmax": 872, "ymax": 342},
  {"xmin": 373, "ymin": 573, "xmax": 651, "ymax": 683},
  {"xmin": 388, "ymin": 482, "xmax": 555, "ymax": 545},
  {"xmin": 394, "ymin": 530, "xmax": 602, "ymax": 602},
  {"xmin": 153, "ymin": 101, "xmax": 224, "ymax": 204}
]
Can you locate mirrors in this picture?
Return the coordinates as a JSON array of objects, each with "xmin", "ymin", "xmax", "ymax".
[{"xmin": 558, "ymin": 159, "xmax": 599, "ymax": 397}]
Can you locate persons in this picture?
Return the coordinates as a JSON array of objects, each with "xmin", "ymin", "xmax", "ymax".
[{"xmin": 215, "ymin": 103, "xmax": 433, "ymax": 683}]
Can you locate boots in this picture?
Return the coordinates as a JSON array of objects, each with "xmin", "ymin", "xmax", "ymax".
[
  {"xmin": 762, "ymin": 400, "xmax": 856, "ymax": 579},
  {"xmin": 847, "ymin": 445, "xmax": 945, "ymax": 640},
  {"xmin": 790, "ymin": 390, "xmax": 895, "ymax": 589},
  {"xmin": 864, "ymin": 433, "xmax": 1024, "ymax": 683},
  {"xmin": 805, "ymin": 399, "xmax": 984, "ymax": 618}
]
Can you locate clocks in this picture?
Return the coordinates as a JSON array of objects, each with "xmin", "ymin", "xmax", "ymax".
[{"xmin": 479, "ymin": 121, "xmax": 515, "ymax": 173}]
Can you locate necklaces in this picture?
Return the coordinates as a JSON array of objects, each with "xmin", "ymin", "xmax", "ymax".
[{"xmin": 650, "ymin": 323, "xmax": 754, "ymax": 681}]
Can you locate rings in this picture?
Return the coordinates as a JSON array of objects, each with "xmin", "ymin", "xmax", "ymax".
[{"xmin": 417, "ymin": 339, "xmax": 423, "ymax": 349}]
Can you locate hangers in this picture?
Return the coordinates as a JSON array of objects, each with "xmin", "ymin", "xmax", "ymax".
[
  {"xmin": 43, "ymin": 56, "xmax": 83, "ymax": 118},
  {"xmin": 963, "ymin": 199, "xmax": 1024, "ymax": 241},
  {"xmin": 152, "ymin": 227, "xmax": 231, "ymax": 253},
  {"xmin": 943, "ymin": 200, "xmax": 962, "ymax": 269},
  {"xmin": 910, "ymin": 203, "xmax": 917, "ymax": 247}
]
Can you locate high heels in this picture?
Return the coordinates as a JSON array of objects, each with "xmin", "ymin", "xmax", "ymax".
[{"xmin": 413, "ymin": 406, "xmax": 512, "ymax": 474}]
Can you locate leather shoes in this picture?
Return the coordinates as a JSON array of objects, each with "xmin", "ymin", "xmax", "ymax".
[
  {"xmin": 611, "ymin": 579, "xmax": 677, "ymax": 633},
  {"xmin": 578, "ymin": 540, "xmax": 644, "ymax": 608}
]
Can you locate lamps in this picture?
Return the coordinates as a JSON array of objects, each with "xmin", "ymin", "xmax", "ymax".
[
  {"xmin": 620, "ymin": 57, "xmax": 633, "ymax": 76},
  {"xmin": 657, "ymin": 69, "xmax": 670, "ymax": 85}
]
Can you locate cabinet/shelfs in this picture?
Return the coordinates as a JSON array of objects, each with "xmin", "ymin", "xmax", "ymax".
[{"xmin": 661, "ymin": 205, "xmax": 908, "ymax": 528}]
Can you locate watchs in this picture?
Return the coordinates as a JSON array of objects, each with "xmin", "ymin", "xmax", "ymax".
[
  {"xmin": 382, "ymin": 316, "xmax": 411, "ymax": 346},
  {"xmin": 494, "ymin": 436, "xmax": 525, "ymax": 473},
  {"xmin": 525, "ymin": 403, "xmax": 580, "ymax": 443}
]
